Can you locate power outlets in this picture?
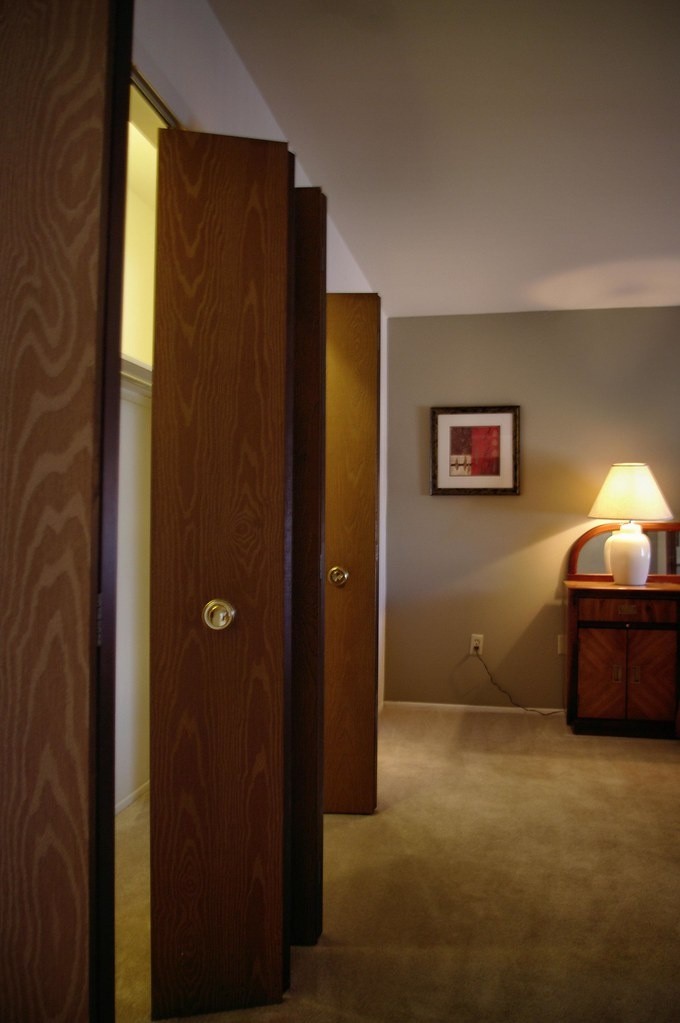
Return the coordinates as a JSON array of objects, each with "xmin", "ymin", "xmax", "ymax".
[
  {"xmin": 469, "ymin": 634, "xmax": 484, "ymax": 656},
  {"xmin": 558, "ymin": 635, "xmax": 566, "ymax": 653}
]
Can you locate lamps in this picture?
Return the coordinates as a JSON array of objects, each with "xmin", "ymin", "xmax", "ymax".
[{"xmin": 588, "ymin": 463, "xmax": 673, "ymax": 586}]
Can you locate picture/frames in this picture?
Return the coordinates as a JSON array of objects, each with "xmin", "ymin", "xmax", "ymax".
[{"xmin": 430, "ymin": 405, "xmax": 521, "ymax": 495}]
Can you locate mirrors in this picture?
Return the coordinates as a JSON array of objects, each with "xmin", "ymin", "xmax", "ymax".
[{"xmin": 567, "ymin": 523, "xmax": 680, "ymax": 584}]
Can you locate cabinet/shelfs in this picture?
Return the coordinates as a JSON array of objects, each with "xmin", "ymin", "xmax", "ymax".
[{"xmin": 564, "ymin": 580, "xmax": 680, "ymax": 740}]
